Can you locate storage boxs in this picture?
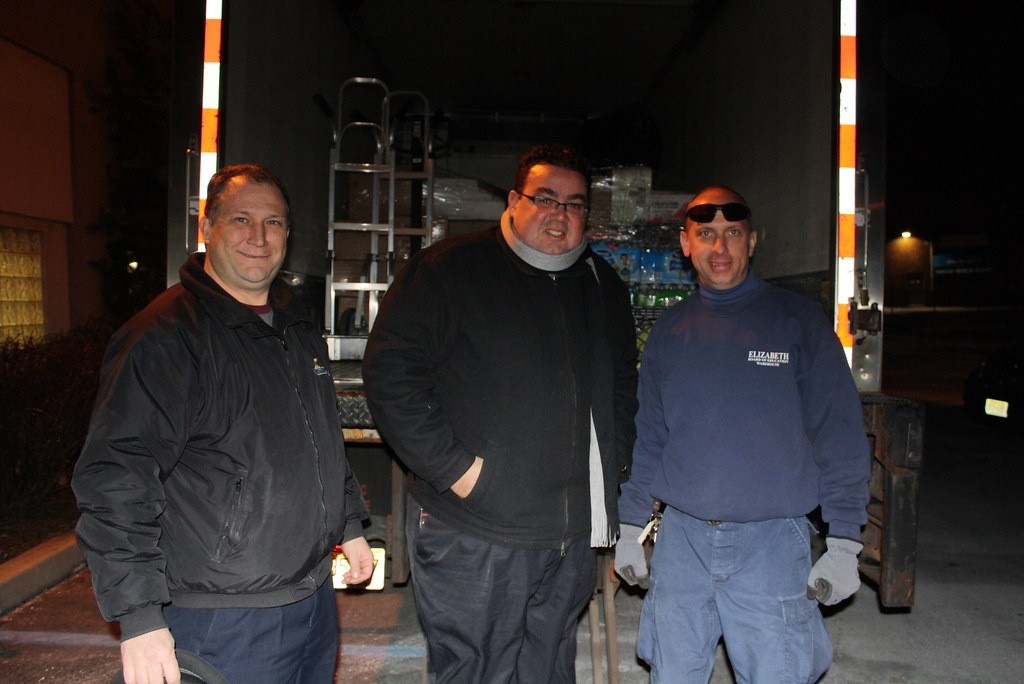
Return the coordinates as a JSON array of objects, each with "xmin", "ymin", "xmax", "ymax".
[{"xmin": 333, "ymin": 160, "xmax": 698, "ymax": 337}]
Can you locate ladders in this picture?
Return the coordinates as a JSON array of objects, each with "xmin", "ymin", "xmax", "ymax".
[{"xmin": 321, "ymin": 74, "xmax": 434, "ymax": 361}]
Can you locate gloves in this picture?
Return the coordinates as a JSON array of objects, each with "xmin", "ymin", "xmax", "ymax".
[
  {"xmin": 615, "ymin": 523, "xmax": 650, "ymax": 589},
  {"xmin": 806, "ymin": 535, "xmax": 865, "ymax": 606}
]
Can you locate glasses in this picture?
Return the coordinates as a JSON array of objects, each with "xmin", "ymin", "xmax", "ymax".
[
  {"xmin": 513, "ymin": 188, "xmax": 587, "ymax": 218},
  {"xmin": 685, "ymin": 203, "xmax": 750, "ymax": 222}
]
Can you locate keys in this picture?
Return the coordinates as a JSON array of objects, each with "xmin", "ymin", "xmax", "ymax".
[{"xmin": 643, "ymin": 507, "xmax": 666, "ymax": 547}]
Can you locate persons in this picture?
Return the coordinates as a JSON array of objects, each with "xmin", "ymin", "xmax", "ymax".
[
  {"xmin": 612, "ymin": 253, "xmax": 635, "ymax": 281},
  {"xmin": 361, "ymin": 147, "xmax": 638, "ymax": 684},
  {"xmin": 71, "ymin": 165, "xmax": 374, "ymax": 684},
  {"xmin": 614, "ymin": 187, "xmax": 872, "ymax": 684}
]
